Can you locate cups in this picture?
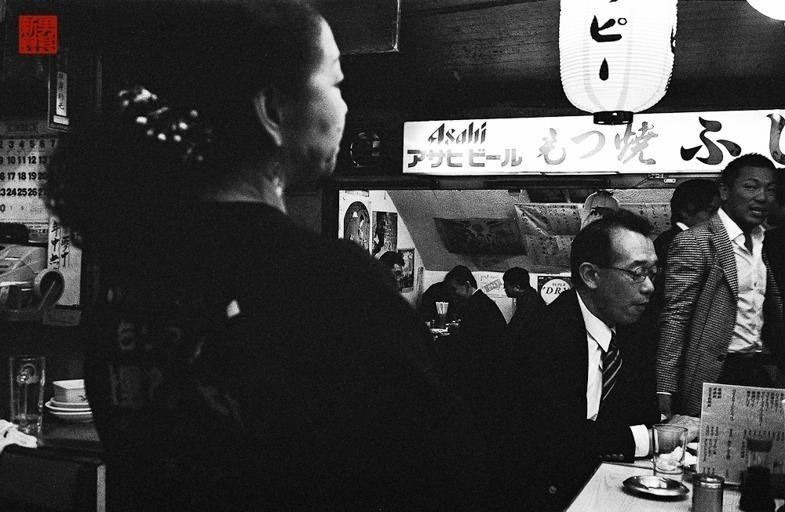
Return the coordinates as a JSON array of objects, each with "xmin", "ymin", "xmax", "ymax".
[
  {"xmin": 8, "ymin": 352, "xmax": 48, "ymax": 436},
  {"xmin": 435, "ymin": 301, "xmax": 449, "ymax": 329},
  {"xmin": 651, "ymin": 424, "xmax": 690, "ymax": 484}
]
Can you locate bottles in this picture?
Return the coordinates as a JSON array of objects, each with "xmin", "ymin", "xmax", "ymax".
[
  {"xmin": 692, "ymin": 473, "xmax": 724, "ymax": 511},
  {"xmin": 743, "ymin": 433, "xmax": 775, "ymax": 468}
]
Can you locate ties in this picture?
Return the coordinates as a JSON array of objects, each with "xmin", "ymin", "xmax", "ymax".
[
  {"xmin": 598, "ymin": 330, "xmax": 626, "ymax": 426},
  {"xmin": 743, "ymin": 229, "xmax": 757, "ymax": 256}
]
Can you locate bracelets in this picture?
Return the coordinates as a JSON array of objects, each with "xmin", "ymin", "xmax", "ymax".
[{"xmin": 652, "ymin": 426, "xmax": 661, "ymax": 457}]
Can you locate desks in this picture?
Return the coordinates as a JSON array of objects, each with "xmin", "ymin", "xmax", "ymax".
[
  {"xmin": 0, "ymin": 421, "xmax": 108, "ymax": 512},
  {"xmin": 563, "ymin": 445, "xmax": 785, "ymax": 512}
]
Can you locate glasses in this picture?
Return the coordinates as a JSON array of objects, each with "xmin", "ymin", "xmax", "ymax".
[{"xmin": 605, "ymin": 263, "xmax": 661, "ymax": 284}]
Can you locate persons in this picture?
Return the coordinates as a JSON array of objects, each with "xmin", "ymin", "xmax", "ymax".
[
  {"xmin": 379, "ymin": 251, "xmax": 404, "ymax": 285},
  {"xmin": 654, "ymin": 155, "xmax": 785, "ymax": 420},
  {"xmin": 45, "ymin": 3, "xmax": 558, "ymax": 510},
  {"xmin": 760, "ymin": 167, "xmax": 785, "ymax": 295},
  {"xmin": 443, "ymin": 265, "xmax": 505, "ymax": 360},
  {"xmin": 416, "ymin": 282, "xmax": 458, "ymax": 321},
  {"xmin": 634, "ymin": 180, "xmax": 723, "ymax": 345},
  {"xmin": 502, "ymin": 266, "xmax": 544, "ymax": 334},
  {"xmin": 500, "ymin": 205, "xmax": 718, "ymax": 498}
]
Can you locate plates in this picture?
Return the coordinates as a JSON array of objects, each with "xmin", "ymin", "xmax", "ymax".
[
  {"xmin": 621, "ymin": 474, "xmax": 690, "ymax": 500},
  {"xmin": 44, "ymin": 397, "xmax": 95, "ymax": 425}
]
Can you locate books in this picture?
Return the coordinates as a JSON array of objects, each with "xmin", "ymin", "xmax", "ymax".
[{"xmin": 698, "ymin": 379, "xmax": 784, "ymax": 485}]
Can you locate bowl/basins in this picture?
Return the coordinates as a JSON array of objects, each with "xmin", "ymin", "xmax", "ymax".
[{"xmin": 51, "ymin": 378, "xmax": 89, "ymax": 406}]
